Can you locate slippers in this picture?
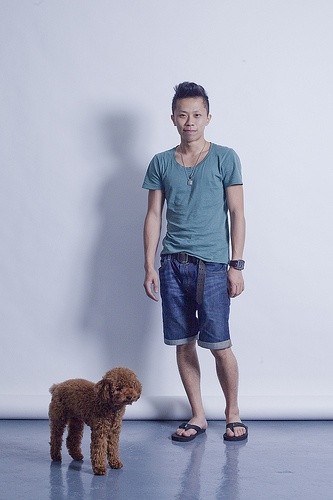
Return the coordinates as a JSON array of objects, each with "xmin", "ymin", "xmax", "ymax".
[
  {"xmin": 223, "ymin": 421, "xmax": 249, "ymax": 441},
  {"xmin": 172, "ymin": 422, "xmax": 206, "ymax": 441}
]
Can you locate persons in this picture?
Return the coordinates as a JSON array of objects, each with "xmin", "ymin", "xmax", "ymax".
[{"xmin": 141, "ymin": 82, "xmax": 249, "ymax": 444}]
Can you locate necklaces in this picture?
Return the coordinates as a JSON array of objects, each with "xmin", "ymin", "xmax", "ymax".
[{"xmin": 180, "ymin": 141, "xmax": 207, "ymax": 186}]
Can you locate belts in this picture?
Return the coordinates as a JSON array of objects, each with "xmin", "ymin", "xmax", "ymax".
[{"xmin": 166, "ymin": 253, "xmax": 205, "ymax": 304}]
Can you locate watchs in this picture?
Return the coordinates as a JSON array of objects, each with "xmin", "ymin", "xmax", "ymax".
[{"xmin": 229, "ymin": 259, "xmax": 245, "ymax": 271}]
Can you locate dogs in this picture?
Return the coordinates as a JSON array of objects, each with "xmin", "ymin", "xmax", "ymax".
[{"xmin": 48, "ymin": 367, "xmax": 142, "ymax": 476}]
